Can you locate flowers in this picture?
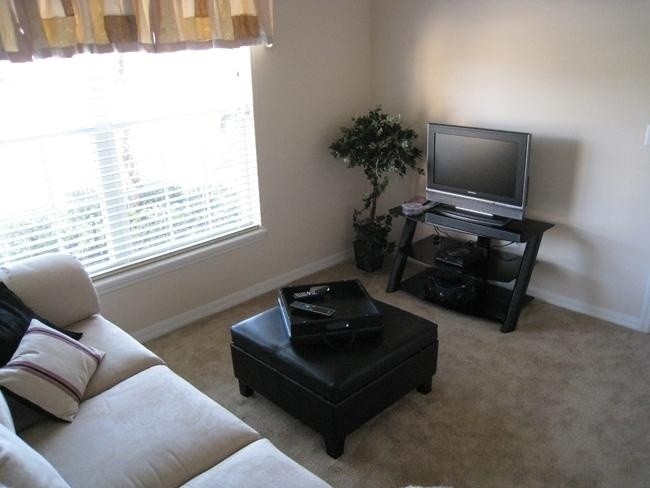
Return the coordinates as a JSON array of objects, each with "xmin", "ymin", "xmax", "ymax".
[{"xmin": 324, "ymin": 105, "xmax": 425, "ymax": 232}]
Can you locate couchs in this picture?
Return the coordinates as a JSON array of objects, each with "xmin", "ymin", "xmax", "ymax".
[{"xmin": 0, "ymin": 238, "xmax": 334, "ymax": 486}]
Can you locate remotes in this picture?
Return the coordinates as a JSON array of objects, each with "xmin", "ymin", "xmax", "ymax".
[
  {"xmin": 288, "ymin": 301, "xmax": 336, "ymax": 317},
  {"xmin": 292, "ymin": 291, "xmax": 321, "ymax": 299}
]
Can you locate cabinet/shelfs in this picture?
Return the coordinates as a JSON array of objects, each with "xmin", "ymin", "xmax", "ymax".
[{"xmin": 383, "ymin": 197, "xmax": 555, "ymax": 338}]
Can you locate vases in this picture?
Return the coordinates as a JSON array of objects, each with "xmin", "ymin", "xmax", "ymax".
[{"xmin": 352, "ymin": 237, "xmax": 393, "ymax": 271}]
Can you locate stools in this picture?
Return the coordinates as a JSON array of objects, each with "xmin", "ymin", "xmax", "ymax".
[{"xmin": 228, "ymin": 288, "xmax": 443, "ymax": 463}]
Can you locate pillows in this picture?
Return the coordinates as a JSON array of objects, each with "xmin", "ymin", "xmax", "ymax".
[
  {"xmin": 0, "ymin": 318, "xmax": 104, "ymax": 431},
  {"xmin": 0, "ymin": 276, "xmax": 87, "ymax": 384}
]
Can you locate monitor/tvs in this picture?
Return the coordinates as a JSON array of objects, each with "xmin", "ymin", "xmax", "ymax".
[{"xmin": 426, "ymin": 123, "xmax": 531, "ymax": 227}]
